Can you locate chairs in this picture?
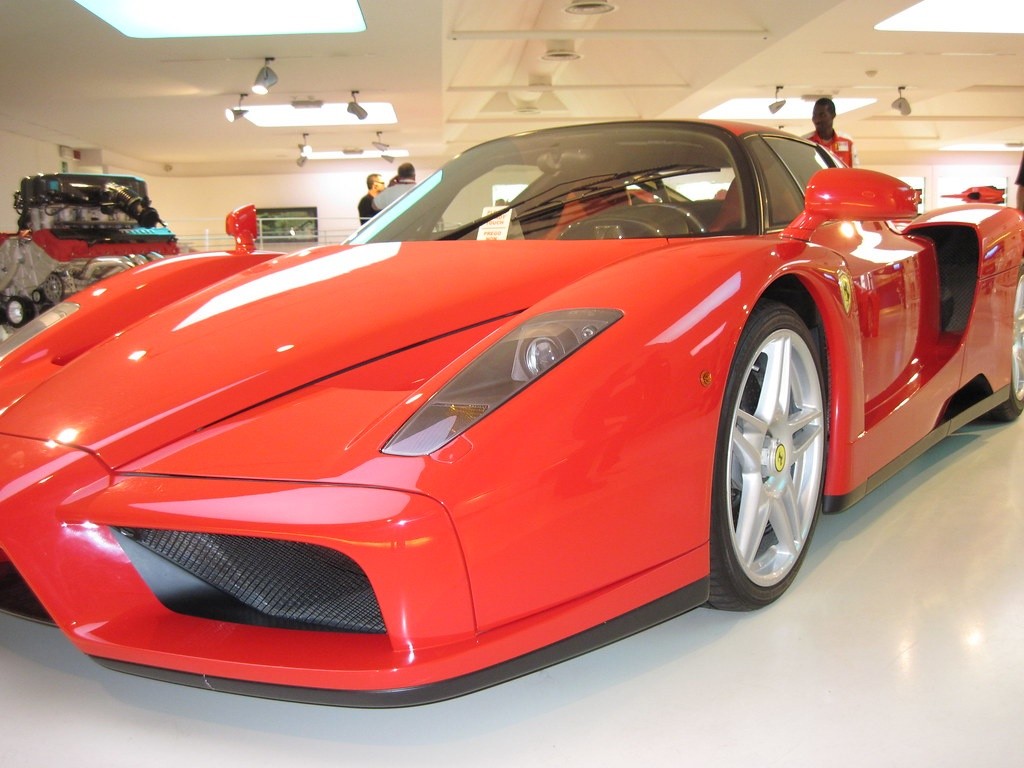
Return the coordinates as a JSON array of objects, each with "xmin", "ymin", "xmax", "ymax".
[{"xmin": 594, "ymin": 203, "xmax": 708, "ymax": 238}]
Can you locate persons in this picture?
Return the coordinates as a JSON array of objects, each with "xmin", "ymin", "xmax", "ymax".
[
  {"xmin": 358, "ymin": 174, "xmax": 384, "ymax": 226},
  {"xmin": 371, "ymin": 163, "xmax": 416, "ymax": 210},
  {"xmin": 800, "ymin": 98, "xmax": 859, "ymax": 168},
  {"xmin": 853, "ymin": 272, "xmax": 879, "ymax": 337},
  {"xmin": 1014, "ymin": 150, "xmax": 1024, "ymax": 211}
]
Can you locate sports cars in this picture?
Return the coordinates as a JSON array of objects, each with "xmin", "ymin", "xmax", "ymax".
[{"xmin": 0, "ymin": 113, "xmax": 1024, "ymax": 710}]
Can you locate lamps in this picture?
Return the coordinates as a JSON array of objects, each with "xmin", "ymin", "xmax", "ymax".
[
  {"xmin": 892, "ymin": 87, "xmax": 911, "ymax": 116},
  {"xmin": 225, "ymin": 94, "xmax": 249, "ymax": 124},
  {"xmin": 296, "ymin": 155, "xmax": 307, "ymax": 168},
  {"xmin": 298, "ymin": 134, "xmax": 313, "ymax": 153},
  {"xmin": 250, "ymin": 57, "xmax": 279, "ymax": 95},
  {"xmin": 564, "ymin": 0, "xmax": 616, "ymax": 17},
  {"xmin": 372, "ymin": 131, "xmax": 390, "ymax": 152},
  {"xmin": 540, "ymin": 41, "xmax": 584, "ymax": 63},
  {"xmin": 515, "ymin": 106, "xmax": 539, "ymax": 113},
  {"xmin": 347, "ymin": 90, "xmax": 368, "ymax": 121},
  {"xmin": 768, "ymin": 85, "xmax": 787, "ymax": 114},
  {"xmin": 381, "ymin": 154, "xmax": 395, "ymax": 164}
]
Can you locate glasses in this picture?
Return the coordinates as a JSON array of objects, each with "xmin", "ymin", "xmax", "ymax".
[{"xmin": 375, "ymin": 181, "xmax": 384, "ymax": 184}]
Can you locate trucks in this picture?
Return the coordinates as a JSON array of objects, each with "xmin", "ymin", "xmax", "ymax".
[{"xmin": 0, "ymin": 170, "xmax": 199, "ymax": 331}]
